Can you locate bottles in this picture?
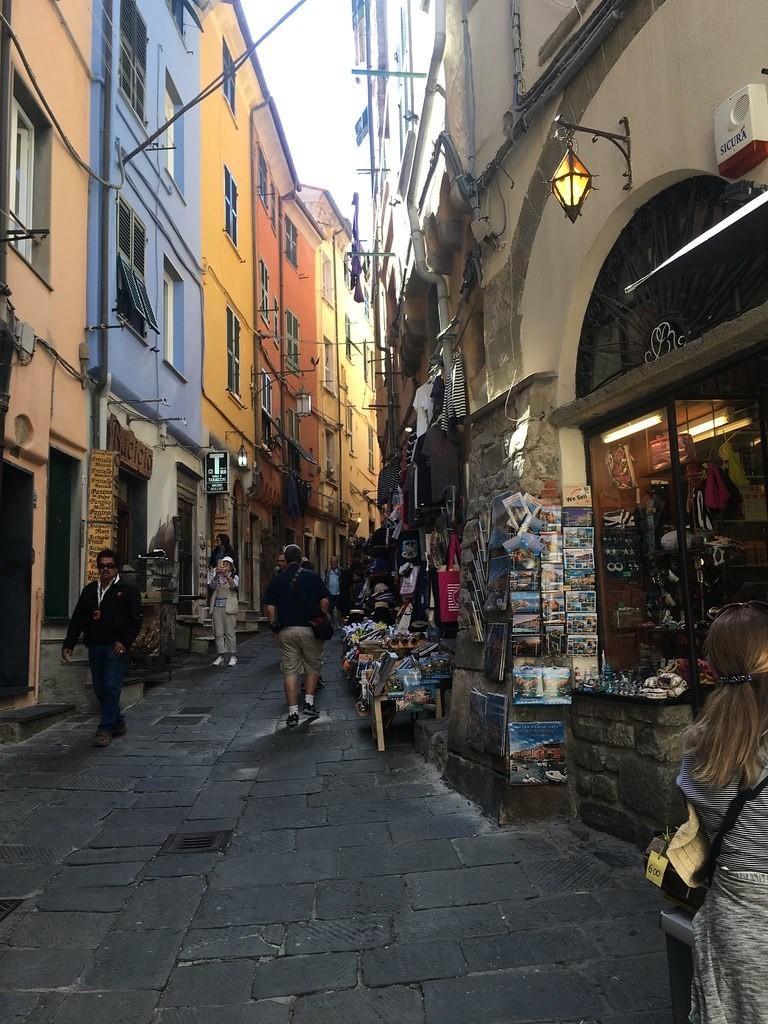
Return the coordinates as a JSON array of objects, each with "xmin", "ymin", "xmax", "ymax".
[
  {"xmin": 585, "ymin": 664, "xmax": 613, "ymax": 694},
  {"xmin": 574, "ymin": 667, "xmax": 580, "ymax": 688},
  {"xmin": 612, "ymin": 665, "xmax": 643, "ymax": 695}
]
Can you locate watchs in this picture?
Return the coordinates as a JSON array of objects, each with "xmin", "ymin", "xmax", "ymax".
[{"xmin": 226, "ymin": 576, "xmax": 231, "ymax": 579}]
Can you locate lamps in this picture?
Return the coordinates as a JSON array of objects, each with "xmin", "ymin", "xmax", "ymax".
[
  {"xmin": 352, "ymin": 512, "xmax": 362, "ymax": 522},
  {"xmin": 551, "ymin": 115, "xmax": 631, "ymax": 222},
  {"xmin": 676, "ymin": 405, "xmax": 737, "ymax": 441},
  {"xmin": 604, "ymin": 414, "xmax": 662, "ymax": 444},
  {"xmin": 224, "ymin": 429, "xmax": 249, "ymax": 466},
  {"xmin": 426, "ymin": 344, "xmax": 464, "ymax": 423},
  {"xmin": 693, "ymin": 415, "xmax": 756, "ymax": 442},
  {"xmin": 250, "ymin": 364, "xmax": 313, "ymax": 417}
]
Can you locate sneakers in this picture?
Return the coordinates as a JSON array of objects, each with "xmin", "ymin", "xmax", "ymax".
[
  {"xmin": 303, "ymin": 701, "xmax": 319, "ymax": 715},
  {"xmin": 228, "ymin": 657, "xmax": 237, "ymax": 665},
  {"xmin": 287, "ymin": 713, "xmax": 298, "ymax": 725},
  {"xmin": 213, "ymin": 656, "xmax": 224, "ymax": 665}
]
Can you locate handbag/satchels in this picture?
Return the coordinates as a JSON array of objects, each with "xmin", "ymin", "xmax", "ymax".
[
  {"xmin": 438, "ymin": 532, "xmax": 462, "ymax": 623},
  {"xmin": 207, "ymin": 568, "xmax": 216, "ymax": 584},
  {"xmin": 307, "ymin": 608, "xmax": 333, "ymax": 640}
]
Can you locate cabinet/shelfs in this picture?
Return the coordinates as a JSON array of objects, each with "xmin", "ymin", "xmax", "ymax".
[{"xmin": 714, "ymin": 519, "xmax": 768, "ymax": 568}]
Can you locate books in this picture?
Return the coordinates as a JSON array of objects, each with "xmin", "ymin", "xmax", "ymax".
[
  {"xmin": 368, "ymin": 640, "xmax": 452, "ymax": 714},
  {"xmin": 464, "ymin": 491, "xmax": 599, "ymax": 785}
]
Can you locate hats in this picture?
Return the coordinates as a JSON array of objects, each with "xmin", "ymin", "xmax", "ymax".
[{"xmin": 223, "ymin": 556, "xmax": 233, "ymax": 565}]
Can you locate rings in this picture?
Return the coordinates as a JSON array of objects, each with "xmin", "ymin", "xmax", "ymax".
[{"xmin": 120, "ymin": 650, "xmax": 122, "ymax": 652}]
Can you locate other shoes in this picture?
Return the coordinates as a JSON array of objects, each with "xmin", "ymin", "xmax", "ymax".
[
  {"xmin": 93, "ymin": 729, "xmax": 112, "ymax": 746},
  {"xmin": 315, "ymin": 676, "xmax": 325, "ymax": 688},
  {"xmin": 301, "ymin": 683, "xmax": 306, "ymax": 690},
  {"xmin": 112, "ymin": 720, "xmax": 127, "ymax": 734}
]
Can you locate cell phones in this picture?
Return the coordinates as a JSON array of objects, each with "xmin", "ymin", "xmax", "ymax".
[{"xmin": 218, "ymin": 559, "xmax": 224, "ymax": 568}]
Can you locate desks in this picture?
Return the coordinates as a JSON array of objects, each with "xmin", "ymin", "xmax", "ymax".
[{"xmin": 366, "ymin": 685, "xmax": 442, "ymax": 752}]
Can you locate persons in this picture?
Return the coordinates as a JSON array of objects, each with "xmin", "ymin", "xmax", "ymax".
[
  {"xmin": 263, "ymin": 545, "xmax": 329, "ymax": 727},
  {"xmin": 208, "ymin": 556, "xmax": 239, "ymax": 667},
  {"xmin": 271, "ymin": 553, "xmax": 361, "ymax": 690},
  {"xmin": 210, "ymin": 533, "xmax": 237, "ymax": 598},
  {"xmin": 676, "ymin": 601, "xmax": 768, "ymax": 1024},
  {"xmin": 61, "ymin": 549, "xmax": 142, "ymax": 747}
]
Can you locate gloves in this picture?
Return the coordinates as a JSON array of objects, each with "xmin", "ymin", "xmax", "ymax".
[{"xmin": 270, "ymin": 621, "xmax": 279, "ymax": 632}]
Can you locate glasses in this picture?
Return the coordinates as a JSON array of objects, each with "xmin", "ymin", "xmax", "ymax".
[
  {"xmin": 97, "ymin": 563, "xmax": 116, "ymax": 569},
  {"xmin": 276, "ymin": 560, "xmax": 285, "ymax": 563},
  {"xmin": 715, "ymin": 601, "xmax": 768, "ymax": 620},
  {"xmin": 215, "ymin": 538, "xmax": 220, "ymax": 542}
]
